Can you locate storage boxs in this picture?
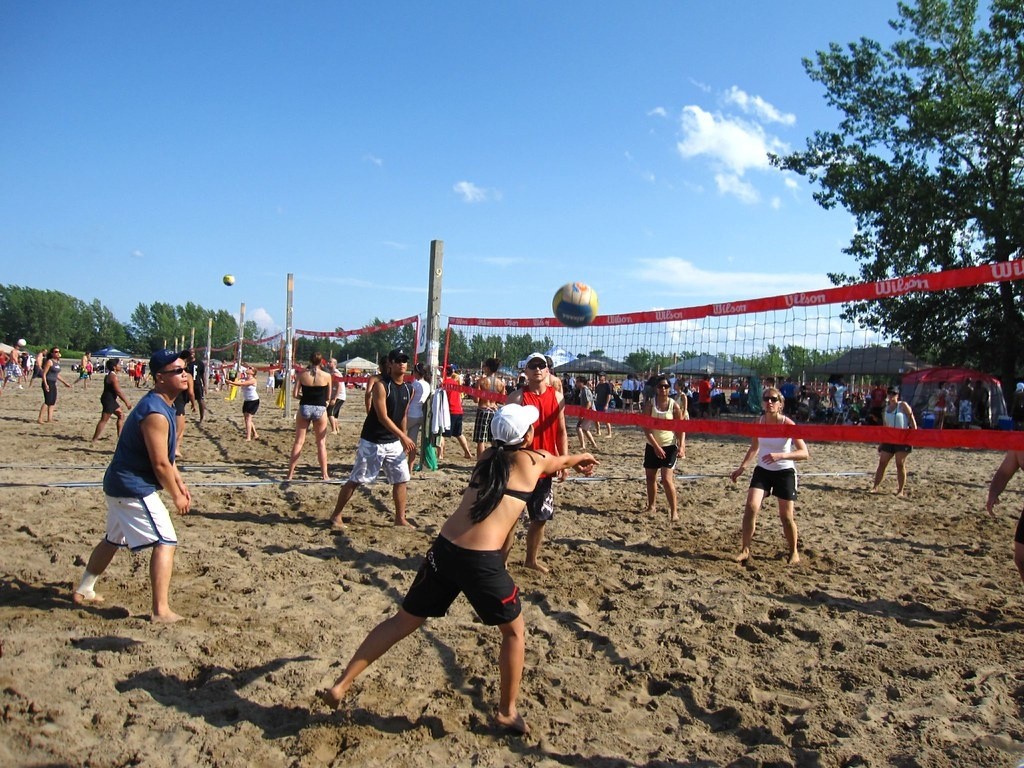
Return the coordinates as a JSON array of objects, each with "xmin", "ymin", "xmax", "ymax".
[
  {"xmin": 922, "ymin": 414, "xmax": 935, "ymax": 429},
  {"xmin": 997, "ymin": 415, "xmax": 1013, "ymax": 430}
]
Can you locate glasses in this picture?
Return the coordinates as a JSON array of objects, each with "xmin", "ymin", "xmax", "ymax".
[
  {"xmin": 159, "ymin": 367, "xmax": 190, "ymax": 374},
  {"xmin": 887, "ymin": 391, "xmax": 898, "ymax": 396},
  {"xmin": 249, "ymin": 367, "xmax": 254, "ymax": 372},
  {"xmin": 55, "ymin": 351, "xmax": 60, "ymax": 353},
  {"xmin": 656, "ymin": 384, "xmax": 670, "ymax": 389},
  {"xmin": 394, "ymin": 358, "xmax": 407, "ymax": 363},
  {"xmin": 764, "ymin": 396, "xmax": 780, "ymax": 403},
  {"xmin": 526, "ymin": 363, "xmax": 546, "ymax": 369}
]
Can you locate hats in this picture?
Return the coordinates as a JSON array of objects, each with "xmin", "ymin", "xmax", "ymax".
[
  {"xmin": 598, "ymin": 372, "xmax": 605, "ymax": 375},
  {"xmin": 525, "ymin": 352, "xmax": 547, "ymax": 365},
  {"xmin": 389, "ymin": 349, "xmax": 410, "ymax": 359},
  {"xmin": 490, "ymin": 404, "xmax": 539, "ymax": 443},
  {"xmin": 149, "ymin": 349, "xmax": 191, "ymax": 371}
]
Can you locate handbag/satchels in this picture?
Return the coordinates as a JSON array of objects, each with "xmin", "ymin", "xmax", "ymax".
[{"xmin": 928, "ymin": 395, "xmax": 938, "ymax": 407}]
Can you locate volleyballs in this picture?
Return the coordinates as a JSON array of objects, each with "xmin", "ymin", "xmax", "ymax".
[
  {"xmin": 552, "ymin": 282, "xmax": 599, "ymax": 328},
  {"xmin": 223, "ymin": 274, "xmax": 235, "ymax": 287},
  {"xmin": 17, "ymin": 338, "xmax": 26, "ymax": 347}
]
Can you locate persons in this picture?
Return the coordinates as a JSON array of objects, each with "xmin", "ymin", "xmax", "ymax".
[
  {"xmin": 316, "ymin": 349, "xmax": 1024, "ymax": 457},
  {"xmin": 0, "ymin": 338, "xmax": 310, "ymax": 443},
  {"xmin": 287, "ymin": 352, "xmax": 331, "ymax": 480},
  {"xmin": 987, "ymin": 432, "xmax": 1024, "ymax": 591},
  {"xmin": 492, "ymin": 353, "xmax": 568, "ymax": 573},
  {"xmin": 74, "ymin": 349, "xmax": 193, "ymax": 624},
  {"xmin": 640, "ymin": 377, "xmax": 682, "ymax": 522},
  {"xmin": 37, "ymin": 347, "xmax": 71, "ymax": 423},
  {"xmin": 324, "ymin": 404, "xmax": 599, "ymax": 734},
  {"xmin": 867, "ymin": 386, "xmax": 917, "ymax": 496},
  {"xmin": 330, "ymin": 348, "xmax": 416, "ymax": 529},
  {"xmin": 730, "ymin": 387, "xmax": 809, "ymax": 564}
]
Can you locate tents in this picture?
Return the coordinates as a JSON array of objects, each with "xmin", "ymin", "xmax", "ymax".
[
  {"xmin": 899, "ymin": 365, "xmax": 1009, "ymax": 431},
  {"xmin": 801, "ymin": 347, "xmax": 936, "ymax": 398},
  {"xmin": 550, "ymin": 356, "xmax": 636, "ymax": 380},
  {"xmin": 663, "ymin": 355, "xmax": 756, "ymax": 381}
]
